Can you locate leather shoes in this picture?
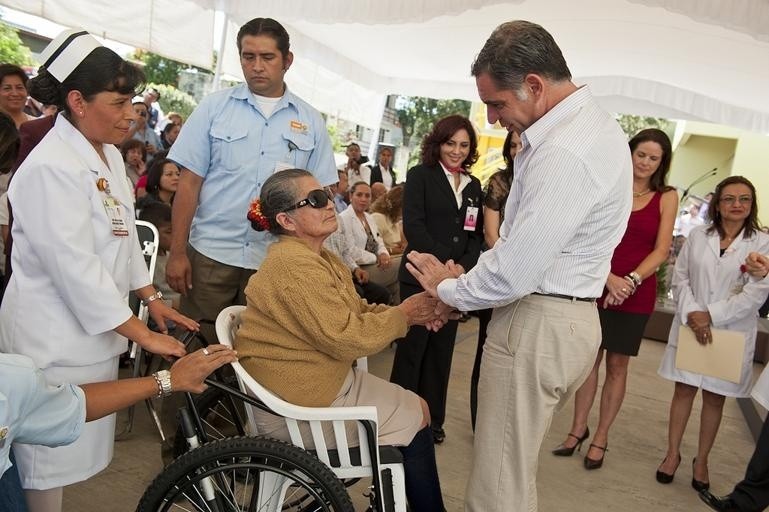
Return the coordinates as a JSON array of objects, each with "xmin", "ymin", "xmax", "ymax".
[{"xmin": 698, "ymin": 488, "xmax": 734, "ymax": 511}]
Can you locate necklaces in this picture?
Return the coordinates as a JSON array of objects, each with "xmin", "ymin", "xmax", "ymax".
[{"xmin": 632, "ymin": 188, "xmax": 652, "ymax": 197}]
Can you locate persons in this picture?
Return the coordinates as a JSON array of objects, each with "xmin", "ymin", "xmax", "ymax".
[
  {"xmin": 163, "ymin": 14, "xmax": 340, "ymax": 497},
  {"xmin": 698, "ymin": 250, "xmax": 769, "ymax": 511},
  {"xmin": 1, "ymin": 345, "xmax": 238, "ymax": 511},
  {"xmin": 655, "ymin": 175, "xmax": 769, "ymax": 492},
  {"xmin": 548, "ymin": 126, "xmax": 678, "ymax": 471},
  {"xmin": 402, "ymin": 16, "xmax": 635, "ymax": 511},
  {"xmin": 1, "ymin": 64, "xmax": 184, "ymax": 330},
  {"xmin": 672, "ymin": 192, "xmax": 769, "ymax": 239},
  {"xmin": 235, "ymin": 168, "xmax": 464, "ymax": 511},
  {"xmin": 471, "ymin": 130, "xmax": 522, "ymax": 434},
  {"xmin": 323, "ymin": 143, "xmax": 406, "ymax": 346},
  {"xmin": 390, "ymin": 115, "xmax": 484, "ymax": 446},
  {"xmin": 1, "ymin": 28, "xmax": 200, "ymax": 512}
]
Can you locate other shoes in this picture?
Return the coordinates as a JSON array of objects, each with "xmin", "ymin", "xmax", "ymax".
[{"xmin": 434, "ymin": 426, "xmax": 447, "ymax": 444}]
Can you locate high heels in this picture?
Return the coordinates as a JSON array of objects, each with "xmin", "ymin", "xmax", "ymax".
[
  {"xmin": 552, "ymin": 426, "xmax": 589, "ymax": 456},
  {"xmin": 587, "ymin": 442, "xmax": 608, "ymax": 469},
  {"xmin": 691, "ymin": 457, "xmax": 710, "ymax": 493},
  {"xmin": 656, "ymin": 453, "xmax": 681, "ymax": 484}
]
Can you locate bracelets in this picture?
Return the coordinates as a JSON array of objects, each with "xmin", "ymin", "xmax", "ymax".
[{"xmin": 623, "ymin": 271, "xmax": 642, "ymax": 289}]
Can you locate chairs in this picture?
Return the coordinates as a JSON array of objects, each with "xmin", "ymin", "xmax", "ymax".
[{"xmin": 120, "ymin": 217, "xmax": 163, "ymax": 440}]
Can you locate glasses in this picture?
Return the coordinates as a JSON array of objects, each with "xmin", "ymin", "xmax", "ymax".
[
  {"xmin": 722, "ymin": 195, "xmax": 753, "ymax": 203},
  {"xmin": 282, "ymin": 186, "xmax": 335, "ymax": 212}
]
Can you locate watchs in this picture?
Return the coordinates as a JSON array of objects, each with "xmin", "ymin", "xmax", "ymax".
[{"xmin": 152, "ymin": 370, "xmax": 172, "ymax": 400}]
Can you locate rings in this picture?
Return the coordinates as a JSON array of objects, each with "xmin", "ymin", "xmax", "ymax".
[
  {"xmin": 703, "ymin": 333, "xmax": 708, "ymax": 338},
  {"xmin": 621, "ymin": 287, "xmax": 627, "ymax": 293},
  {"xmin": 202, "ymin": 347, "xmax": 209, "ymax": 355}
]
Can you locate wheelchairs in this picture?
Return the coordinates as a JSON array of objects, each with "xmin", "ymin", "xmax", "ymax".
[{"xmin": 131, "ymin": 305, "xmax": 408, "ymax": 512}]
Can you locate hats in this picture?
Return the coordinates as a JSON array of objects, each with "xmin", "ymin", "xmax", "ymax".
[{"xmin": 37, "ymin": 27, "xmax": 104, "ymax": 84}]
